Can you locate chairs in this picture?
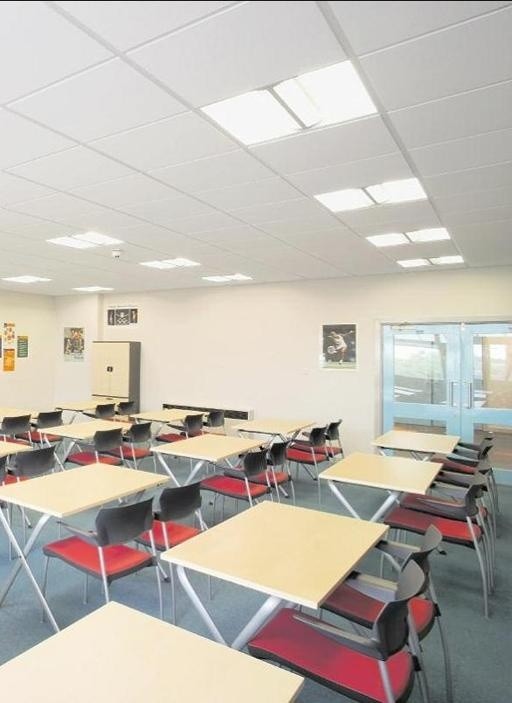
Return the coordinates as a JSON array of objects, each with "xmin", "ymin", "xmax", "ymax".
[
  {"xmin": 246, "ymin": 554, "xmax": 427, "ymax": 703},
  {"xmin": 322, "ymin": 524, "xmax": 453, "ymax": 703}
]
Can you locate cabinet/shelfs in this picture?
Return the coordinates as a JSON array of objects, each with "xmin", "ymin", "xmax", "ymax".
[{"xmin": 88, "ymin": 341, "xmax": 140, "ymax": 415}]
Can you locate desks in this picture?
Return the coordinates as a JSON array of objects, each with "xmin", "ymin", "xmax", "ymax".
[
  {"xmin": 2, "ymin": 599, "xmax": 308, "ymax": 703},
  {"xmin": 160, "ymin": 500, "xmax": 390, "ymax": 651}
]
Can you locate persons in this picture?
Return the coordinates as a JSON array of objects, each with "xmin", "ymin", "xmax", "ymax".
[{"xmin": 325, "ymin": 329, "xmax": 355, "ymax": 365}]
